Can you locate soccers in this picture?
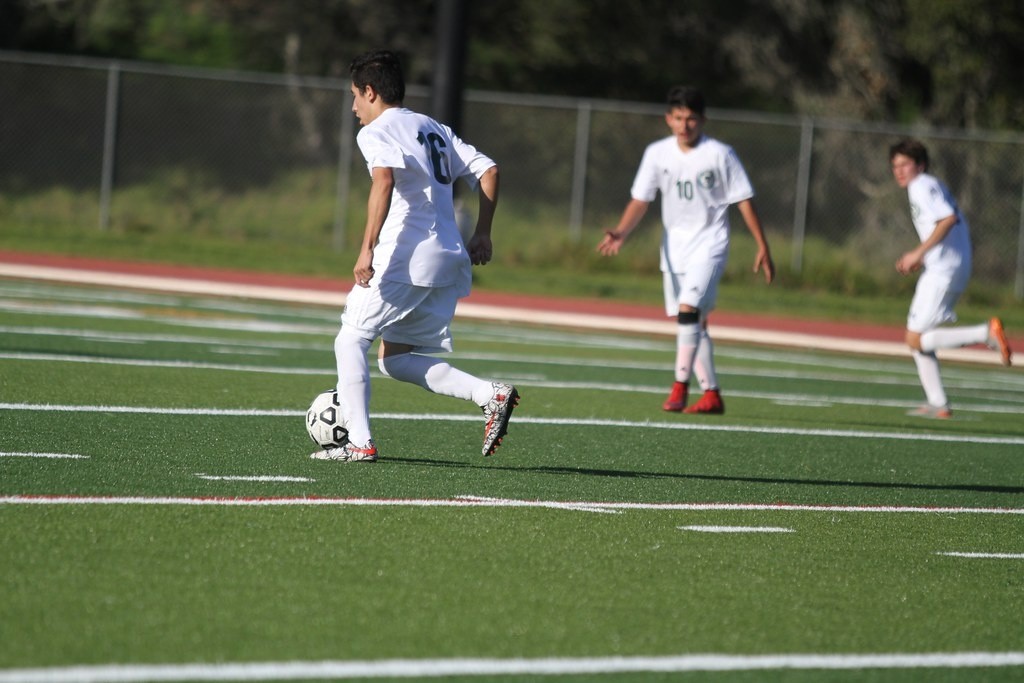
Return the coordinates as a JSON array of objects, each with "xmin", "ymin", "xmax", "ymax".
[{"xmin": 305, "ymin": 388, "xmax": 349, "ymax": 450}]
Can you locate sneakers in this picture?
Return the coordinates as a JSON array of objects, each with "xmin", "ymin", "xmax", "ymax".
[
  {"xmin": 983, "ymin": 316, "xmax": 1012, "ymax": 369},
  {"xmin": 310, "ymin": 438, "xmax": 378, "ymax": 462},
  {"xmin": 663, "ymin": 381, "xmax": 689, "ymax": 411},
  {"xmin": 906, "ymin": 403, "xmax": 953, "ymax": 418},
  {"xmin": 480, "ymin": 381, "xmax": 521, "ymax": 457},
  {"xmin": 682, "ymin": 388, "xmax": 724, "ymax": 414}
]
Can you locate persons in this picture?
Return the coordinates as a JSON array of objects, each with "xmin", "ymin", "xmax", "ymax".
[
  {"xmin": 891, "ymin": 139, "xmax": 1011, "ymax": 421},
  {"xmin": 310, "ymin": 52, "xmax": 521, "ymax": 464},
  {"xmin": 595, "ymin": 86, "xmax": 774, "ymax": 414}
]
